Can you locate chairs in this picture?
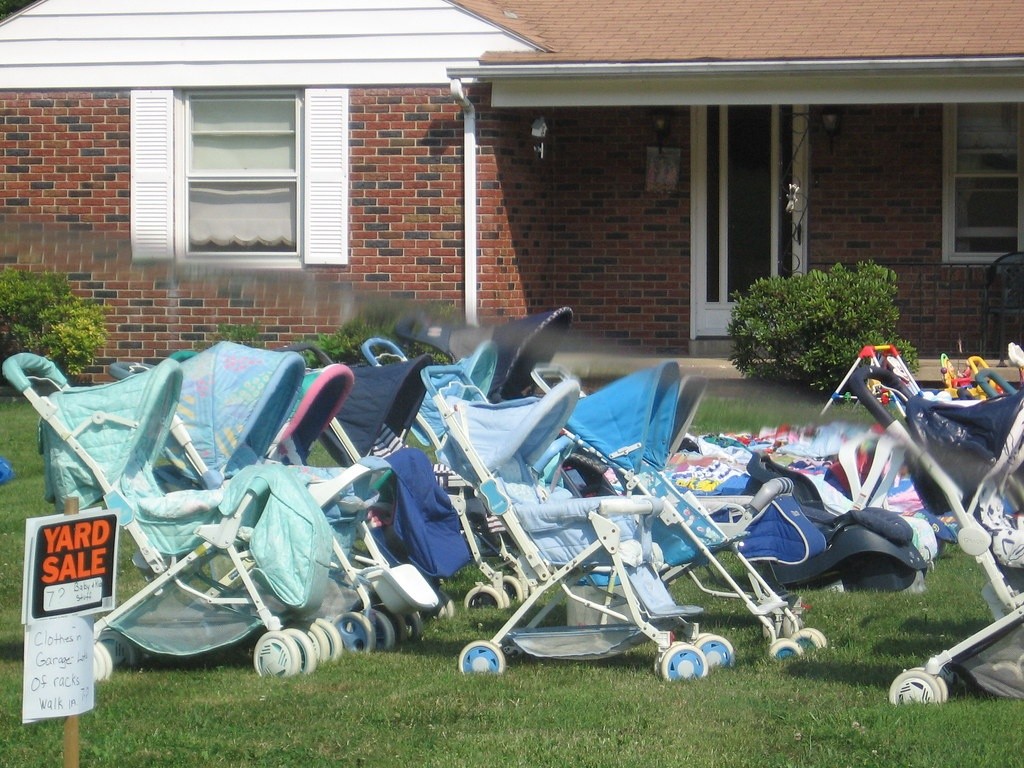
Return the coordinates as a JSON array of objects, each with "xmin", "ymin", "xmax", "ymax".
[{"xmin": 981, "ymin": 249, "xmax": 1024, "ymax": 358}]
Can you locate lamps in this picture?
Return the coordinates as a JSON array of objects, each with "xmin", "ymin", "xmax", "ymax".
[
  {"xmin": 651, "ymin": 106, "xmax": 670, "ymax": 154},
  {"xmin": 821, "ymin": 105, "xmax": 840, "ymax": 156}
]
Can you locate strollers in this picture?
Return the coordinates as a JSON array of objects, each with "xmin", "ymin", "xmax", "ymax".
[{"xmin": 0, "ymin": 308, "xmax": 1023, "ymax": 709}]
